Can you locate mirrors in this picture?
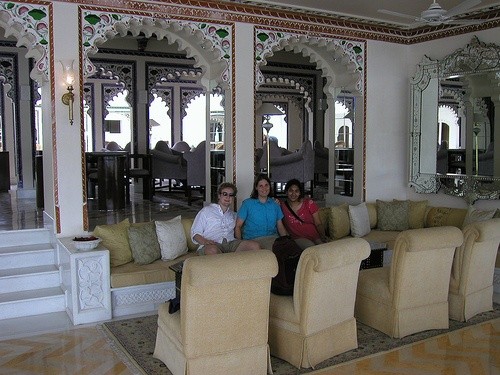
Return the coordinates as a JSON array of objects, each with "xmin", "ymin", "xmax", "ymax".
[{"xmin": 407, "ymin": 35, "xmax": 500, "ymax": 205}]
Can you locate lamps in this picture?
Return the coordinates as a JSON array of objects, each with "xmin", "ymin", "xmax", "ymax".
[
  {"xmin": 60, "ymin": 59, "xmax": 76, "ymax": 125},
  {"xmin": 473, "ymin": 112, "xmax": 486, "ymax": 175},
  {"xmin": 256, "ymin": 103, "xmax": 284, "ymax": 178},
  {"xmin": 149, "ymin": 119, "xmax": 160, "ymax": 137}
]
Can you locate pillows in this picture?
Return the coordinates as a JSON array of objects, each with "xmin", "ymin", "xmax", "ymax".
[
  {"xmin": 318, "ymin": 198, "xmax": 500, "ymax": 240},
  {"xmin": 94, "ymin": 214, "xmax": 196, "ymax": 267}
]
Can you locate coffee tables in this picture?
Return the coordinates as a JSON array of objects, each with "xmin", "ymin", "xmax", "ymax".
[{"xmin": 168, "ymin": 241, "xmax": 388, "ymax": 303}]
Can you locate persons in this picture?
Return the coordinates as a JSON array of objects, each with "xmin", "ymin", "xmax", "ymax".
[
  {"xmin": 271, "ymin": 178, "xmax": 325, "ymax": 250},
  {"xmin": 190, "ymin": 182, "xmax": 261, "ymax": 255},
  {"xmin": 235, "ymin": 176, "xmax": 289, "ymax": 250}
]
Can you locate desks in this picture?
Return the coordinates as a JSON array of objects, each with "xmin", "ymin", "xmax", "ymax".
[{"xmin": 85, "ymin": 152, "xmax": 126, "ymax": 211}]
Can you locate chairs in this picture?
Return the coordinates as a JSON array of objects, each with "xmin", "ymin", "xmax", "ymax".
[{"xmin": 85, "ymin": 139, "xmax": 328, "ymax": 206}]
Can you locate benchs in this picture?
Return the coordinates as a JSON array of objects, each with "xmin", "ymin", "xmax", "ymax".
[{"xmin": 110, "ymin": 206, "xmax": 500, "ymax": 317}]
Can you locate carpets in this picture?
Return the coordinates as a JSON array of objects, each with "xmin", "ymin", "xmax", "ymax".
[{"xmin": 94, "ymin": 302, "xmax": 500, "ymax": 375}]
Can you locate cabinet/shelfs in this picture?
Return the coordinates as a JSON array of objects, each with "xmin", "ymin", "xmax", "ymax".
[{"xmin": 0, "ymin": 151, "xmax": 10, "ymax": 193}]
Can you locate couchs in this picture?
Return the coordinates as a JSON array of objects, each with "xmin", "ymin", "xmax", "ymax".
[
  {"xmin": 355, "ymin": 226, "xmax": 463, "ymax": 339},
  {"xmin": 268, "ymin": 238, "xmax": 371, "ymax": 370},
  {"xmin": 448, "ymin": 218, "xmax": 500, "ymax": 321},
  {"xmin": 153, "ymin": 248, "xmax": 278, "ymax": 375},
  {"xmin": 478, "ymin": 147, "xmax": 494, "ymax": 176}
]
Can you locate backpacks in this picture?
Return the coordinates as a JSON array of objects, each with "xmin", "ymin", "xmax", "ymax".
[{"xmin": 271, "ymin": 236, "xmax": 303, "ymax": 295}]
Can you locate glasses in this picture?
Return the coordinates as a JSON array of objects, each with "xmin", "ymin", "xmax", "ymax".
[{"xmin": 220, "ymin": 192, "xmax": 234, "ymax": 196}]
